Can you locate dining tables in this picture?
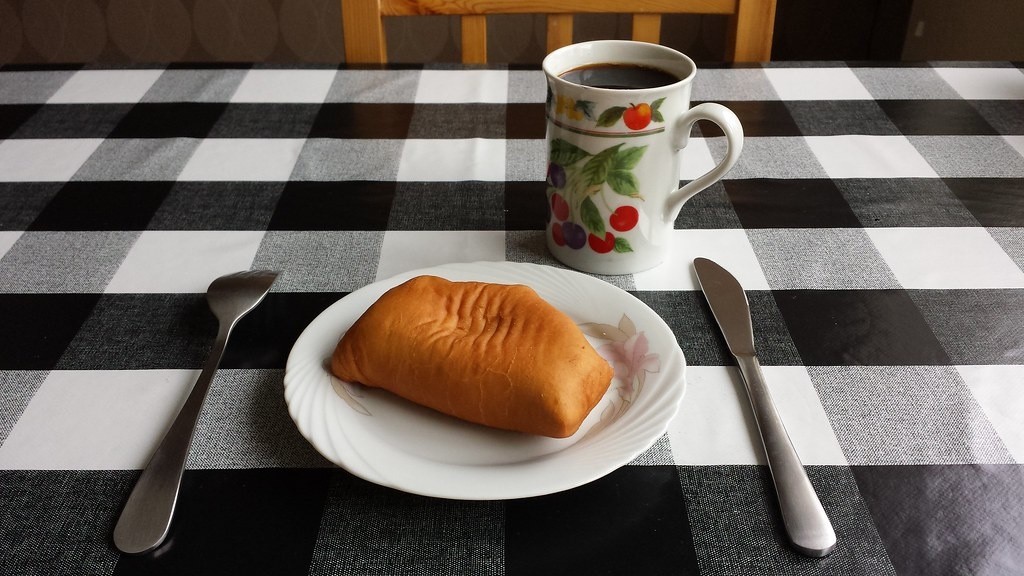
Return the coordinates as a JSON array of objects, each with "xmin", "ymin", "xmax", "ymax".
[{"xmin": 0, "ymin": 68, "xmax": 1024, "ymax": 576}]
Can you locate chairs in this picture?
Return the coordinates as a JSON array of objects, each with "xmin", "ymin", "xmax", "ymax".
[{"xmin": 339, "ymin": 0, "xmax": 778, "ymax": 68}]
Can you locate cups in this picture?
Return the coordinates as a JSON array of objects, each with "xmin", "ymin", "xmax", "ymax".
[{"xmin": 543, "ymin": 39, "xmax": 744, "ymax": 276}]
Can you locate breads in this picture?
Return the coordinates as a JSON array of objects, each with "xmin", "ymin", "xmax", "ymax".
[{"xmin": 332, "ymin": 273, "xmax": 614, "ymax": 441}]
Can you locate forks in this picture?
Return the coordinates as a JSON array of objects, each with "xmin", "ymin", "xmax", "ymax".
[{"xmin": 112, "ymin": 269, "xmax": 281, "ymax": 552}]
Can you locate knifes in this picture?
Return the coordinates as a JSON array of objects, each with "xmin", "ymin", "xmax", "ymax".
[{"xmin": 693, "ymin": 255, "xmax": 838, "ymax": 555}]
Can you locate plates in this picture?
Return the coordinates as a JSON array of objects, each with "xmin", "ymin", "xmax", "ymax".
[{"xmin": 283, "ymin": 260, "xmax": 686, "ymax": 501}]
